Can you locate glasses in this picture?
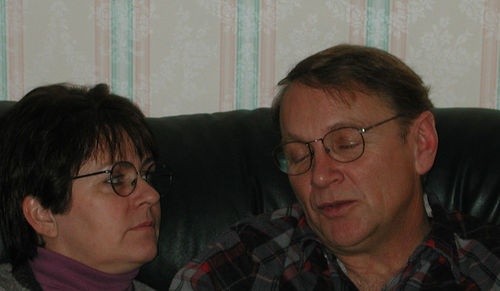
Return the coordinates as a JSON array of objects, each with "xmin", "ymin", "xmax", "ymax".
[
  {"xmin": 272, "ymin": 113, "xmax": 412, "ymax": 176},
  {"xmin": 68, "ymin": 161, "xmax": 177, "ymax": 201}
]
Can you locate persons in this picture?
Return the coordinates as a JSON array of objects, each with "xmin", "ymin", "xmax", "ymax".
[
  {"xmin": 0, "ymin": 81, "xmax": 177, "ymax": 291},
  {"xmin": 168, "ymin": 44, "xmax": 500, "ymax": 291}
]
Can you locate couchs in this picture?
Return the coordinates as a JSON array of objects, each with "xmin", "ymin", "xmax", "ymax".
[{"xmin": 0, "ymin": 100, "xmax": 500, "ymax": 291}]
